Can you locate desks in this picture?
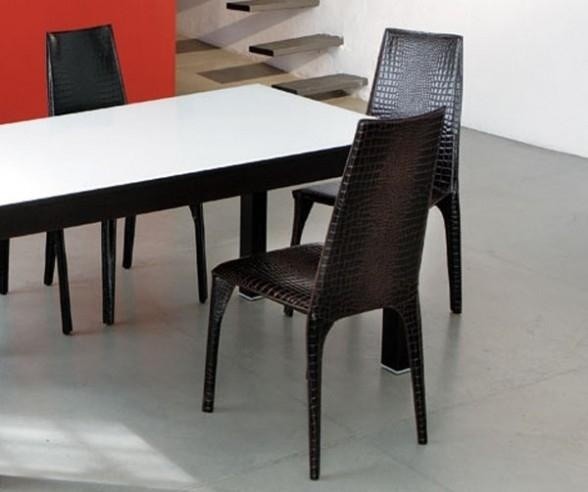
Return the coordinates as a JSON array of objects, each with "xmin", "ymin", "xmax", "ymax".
[{"xmin": 0, "ymin": 78, "xmax": 381, "ymax": 329}]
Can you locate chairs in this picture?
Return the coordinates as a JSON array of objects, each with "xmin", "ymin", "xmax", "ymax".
[{"xmin": 36, "ymin": 20, "xmax": 218, "ymax": 338}]
